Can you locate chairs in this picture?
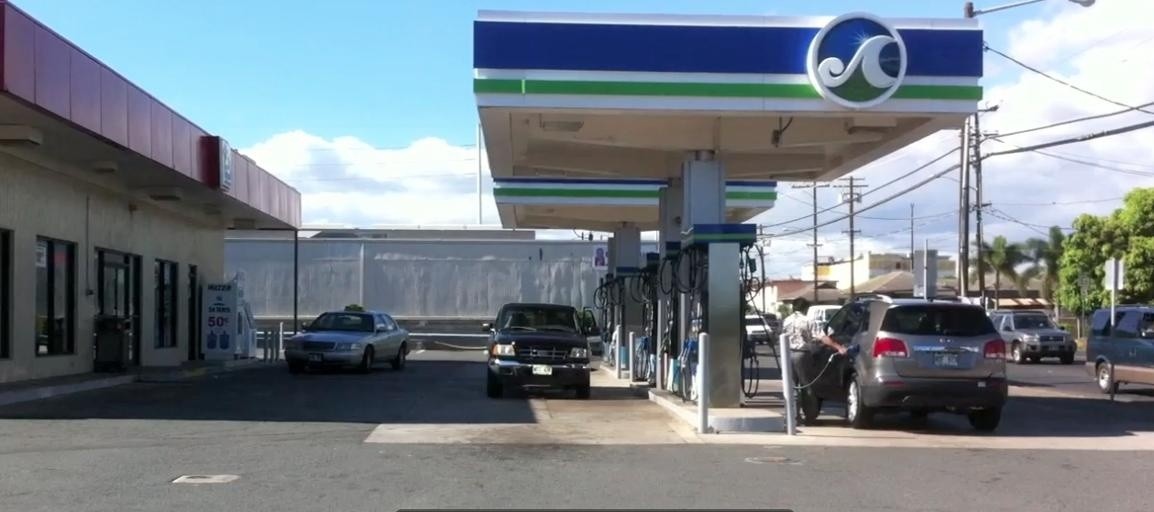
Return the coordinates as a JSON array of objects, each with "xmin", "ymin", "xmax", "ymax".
[{"xmin": 503, "ymin": 313, "xmax": 529, "ymax": 330}]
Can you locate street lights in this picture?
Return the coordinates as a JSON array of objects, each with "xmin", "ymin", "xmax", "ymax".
[{"xmin": 953, "ymin": 0, "xmax": 1100, "ymax": 299}]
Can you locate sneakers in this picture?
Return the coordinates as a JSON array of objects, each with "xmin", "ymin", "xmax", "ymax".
[{"xmin": 804, "ymin": 420, "xmax": 825, "ymax": 426}]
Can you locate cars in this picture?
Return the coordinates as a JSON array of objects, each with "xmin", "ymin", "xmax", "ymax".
[
  {"xmin": 691, "ymin": 318, "xmax": 702, "ymax": 333},
  {"xmin": 282, "ymin": 302, "xmax": 412, "ymax": 370}
]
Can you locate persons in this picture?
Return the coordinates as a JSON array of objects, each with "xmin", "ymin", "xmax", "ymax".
[{"xmin": 782, "ymin": 297, "xmax": 849, "ymax": 427}]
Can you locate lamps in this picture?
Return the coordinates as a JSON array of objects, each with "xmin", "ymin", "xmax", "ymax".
[
  {"xmin": 840, "ymin": 111, "xmax": 899, "ymax": 134},
  {"xmin": 537, "ymin": 111, "xmax": 586, "ymax": 133}
]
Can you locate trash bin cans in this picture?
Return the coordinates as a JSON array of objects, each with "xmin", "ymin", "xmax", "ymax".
[{"xmin": 95, "ymin": 314, "xmax": 132, "ymax": 373}]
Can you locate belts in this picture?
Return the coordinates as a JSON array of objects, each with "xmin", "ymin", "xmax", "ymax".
[{"xmin": 790, "ymin": 349, "xmax": 810, "ymax": 352}]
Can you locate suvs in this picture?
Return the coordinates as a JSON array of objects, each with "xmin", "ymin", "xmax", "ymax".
[{"xmin": 479, "ymin": 301, "xmax": 599, "ymax": 401}]
